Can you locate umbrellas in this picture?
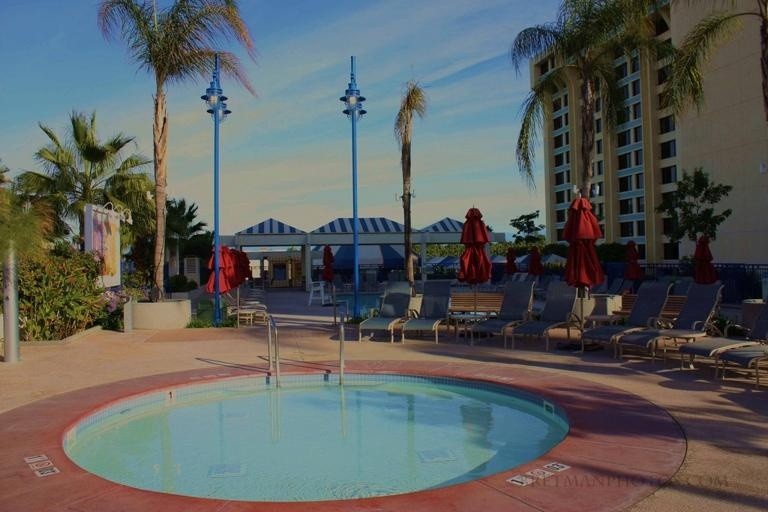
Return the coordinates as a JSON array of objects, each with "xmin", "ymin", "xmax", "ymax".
[
  {"xmin": 206, "ymin": 245, "xmax": 250, "ymax": 318},
  {"xmin": 624, "ymin": 239, "xmax": 644, "ymax": 292},
  {"xmin": 527, "ymin": 245, "xmax": 545, "ymax": 284},
  {"xmin": 320, "ymin": 244, "xmax": 340, "ymax": 305},
  {"xmin": 458, "ymin": 206, "xmax": 496, "ymax": 322},
  {"xmin": 690, "ymin": 230, "xmax": 719, "ymax": 286},
  {"xmin": 562, "ymin": 195, "xmax": 604, "ymax": 353},
  {"xmin": 504, "ymin": 246, "xmax": 518, "ymax": 275}
]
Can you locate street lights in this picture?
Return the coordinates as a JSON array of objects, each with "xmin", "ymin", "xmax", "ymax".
[
  {"xmin": 338, "ymin": 51, "xmax": 369, "ymax": 325},
  {"xmin": 202, "ymin": 49, "xmax": 231, "ymax": 328}
]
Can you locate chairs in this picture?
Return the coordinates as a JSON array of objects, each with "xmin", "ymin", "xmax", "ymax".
[{"xmin": 222, "ymin": 288, "xmax": 269, "ymax": 326}]
